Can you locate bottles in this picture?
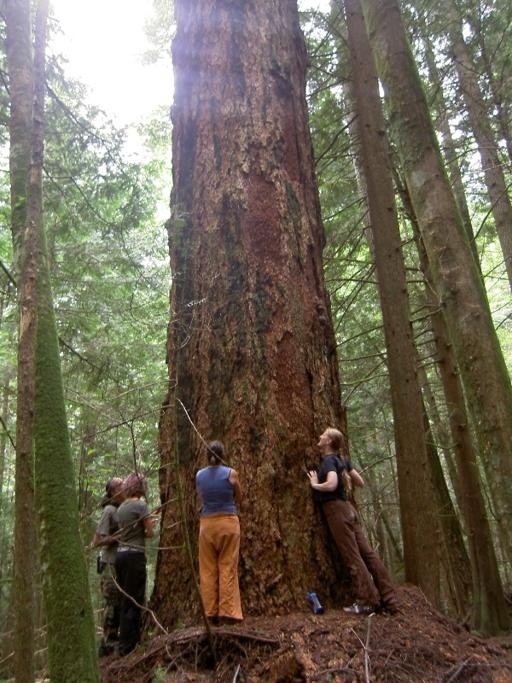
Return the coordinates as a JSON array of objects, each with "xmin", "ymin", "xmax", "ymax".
[{"xmin": 308, "ymin": 592, "xmax": 324, "ymax": 614}]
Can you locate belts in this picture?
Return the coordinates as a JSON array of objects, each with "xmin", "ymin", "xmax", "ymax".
[{"xmin": 116, "ymin": 547, "xmax": 143, "ymax": 553}]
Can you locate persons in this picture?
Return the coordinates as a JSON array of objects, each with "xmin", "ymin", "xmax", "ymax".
[
  {"xmin": 194, "ymin": 440, "xmax": 244, "ymax": 625},
  {"xmin": 94, "ymin": 476, "xmax": 126, "ymax": 657},
  {"xmin": 306, "ymin": 426, "xmax": 399, "ymax": 616},
  {"xmin": 115, "ymin": 473, "xmax": 154, "ymax": 658}
]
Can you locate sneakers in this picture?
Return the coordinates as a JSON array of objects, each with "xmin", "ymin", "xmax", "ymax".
[{"xmin": 343, "ymin": 604, "xmax": 380, "ymax": 615}]
